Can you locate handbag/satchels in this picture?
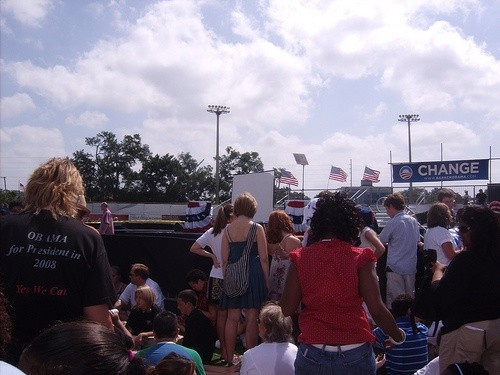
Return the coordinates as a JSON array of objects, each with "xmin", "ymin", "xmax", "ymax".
[
  {"xmin": 224, "ymin": 256, "xmax": 244, "ymax": 297},
  {"xmin": 268, "ymin": 254, "xmax": 290, "ymax": 294}
]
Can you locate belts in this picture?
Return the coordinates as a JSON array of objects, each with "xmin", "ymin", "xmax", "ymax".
[{"xmin": 309, "ymin": 342, "xmax": 364, "ymax": 353}]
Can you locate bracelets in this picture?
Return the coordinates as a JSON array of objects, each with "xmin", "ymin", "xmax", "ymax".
[{"xmin": 389, "ymin": 328, "xmax": 407, "ymax": 345}]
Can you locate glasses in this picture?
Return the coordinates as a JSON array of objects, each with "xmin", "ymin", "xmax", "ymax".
[
  {"xmin": 444, "ymin": 199, "xmax": 455, "ymax": 205},
  {"xmin": 459, "ymin": 226, "xmax": 471, "ymax": 233}
]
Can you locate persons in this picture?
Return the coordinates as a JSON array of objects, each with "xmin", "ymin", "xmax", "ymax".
[
  {"xmin": 0, "ymin": 309, "xmax": 205, "ymax": 375},
  {"xmin": 240, "ymin": 304, "xmax": 298, "ymax": 375},
  {"xmin": 423, "ymin": 188, "xmax": 500, "ymax": 375},
  {"xmin": 124, "ymin": 285, "xmax": 162, "ymax": 338},
  {"xmin": 378, "ymin": 193, "xmax": 421, "ymax": 311},
  {"xmin": 176, "ymin": 289, "xmax": 215, "ymax": 362},
  {"xmin": 187, "ymin": 270, "xmax": 216, "ymax": 318},
  {"xmin": 99, "ymin": 202, "xmax": 114, "ymax": 235},
  {"xmin": 8, "ymin": 200, "xmax": 25, "ymax": 215},
  {"xmin": 192, "ymin": 192, "xmax": 330, "ymax": 367},
  {"xmin": 114, "ymin": 264, "xmax": 165, "ymax": 313},
  {"xmin": 0, "ymin": 158, "xmax": 116, "ymax": 362},
  {"xmin": 282, "ymin": 192, "xmax": 403, "ymax": 375},
  {"xmin": 373, "ymin": 294, "xmax": 428, "ymax": 375},
  {"xmin": 355, "ymin": 208, "xmax": 385, "ymax": 260}
]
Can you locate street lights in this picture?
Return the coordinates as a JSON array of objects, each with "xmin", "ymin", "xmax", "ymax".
[
  {"xmin": 206, "ymin": 105, "xmax": 231, "ymax": 205},
  {"xmin": 397, "ymin": 113, "xmax": 421, "ymax": 190}
]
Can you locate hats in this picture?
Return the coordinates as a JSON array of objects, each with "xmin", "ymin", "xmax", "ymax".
[
  {"xmin": 490, "ymin": 201, "xmax": 500, "ymax": 209},
  {"xmin": 357, "ymin": 208, "xmax": 372, "ymax": 223}
]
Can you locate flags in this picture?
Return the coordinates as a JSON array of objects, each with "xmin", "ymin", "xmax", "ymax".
[
  {"xmin": 362, "ymin": 168, "xmax": 380, "ymax": 182},
  {"xmin": 329, "ymin": 167, "xmax": 348, "ymax": 182},
  {"xmin": 279, "ymin": 170, "xmax": 298, "ymax": 186}
]
